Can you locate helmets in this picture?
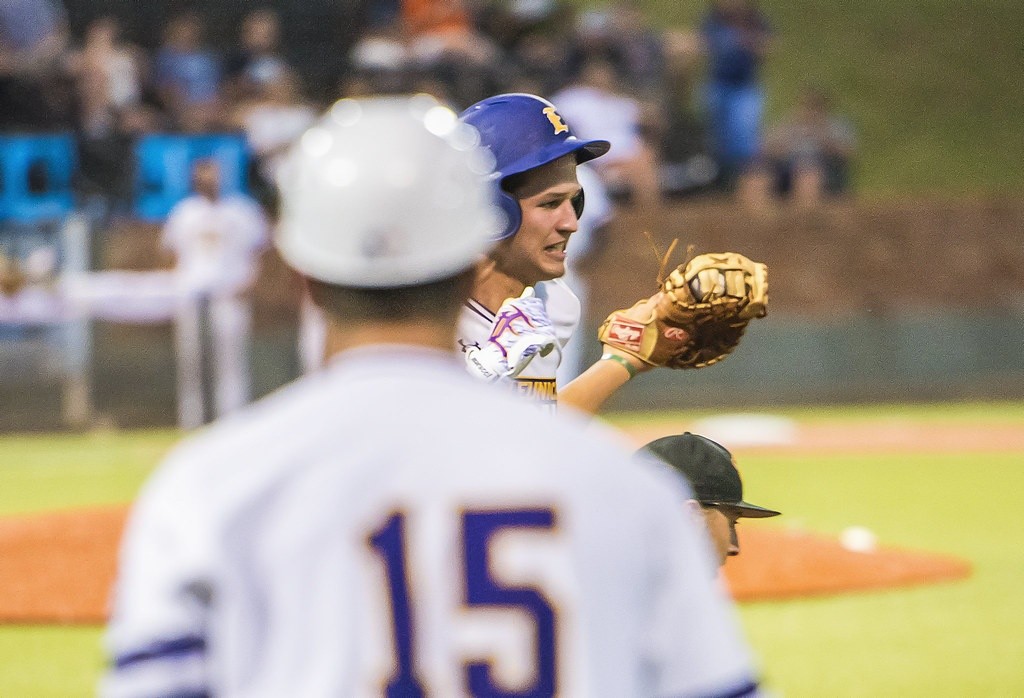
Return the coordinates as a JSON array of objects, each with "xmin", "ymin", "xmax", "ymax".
[
  {"xmin": 275, "ymin": 95, "xmax": 506, "ymax": 287},
  {"xmin": 457, "ymin": 93, "xmax": 611, "ymax": 242}
]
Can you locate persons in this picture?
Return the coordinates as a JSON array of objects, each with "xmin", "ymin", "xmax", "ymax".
[
  {"xmin": 100, "ymin": 91, "xmax": 780, "ymax": 697},
  {"xmin": 0, "ymin": 0, "xmax": 851, "ymax": 217}
]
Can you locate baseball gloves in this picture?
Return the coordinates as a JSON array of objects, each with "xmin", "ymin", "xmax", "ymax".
[{"xmin": 593, "ymin": 230, "xmax": 774, "ymax": 374}]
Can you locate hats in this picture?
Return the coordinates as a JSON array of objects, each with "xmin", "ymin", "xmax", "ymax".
[{"xmin": 630, "ymin": 431, "xmax": 782, "ymax": 518}]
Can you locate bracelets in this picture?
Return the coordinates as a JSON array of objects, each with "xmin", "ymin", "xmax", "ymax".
[{"xmin": 601, "ymin": 353, "xmax": 635, "ymax": 380}]
